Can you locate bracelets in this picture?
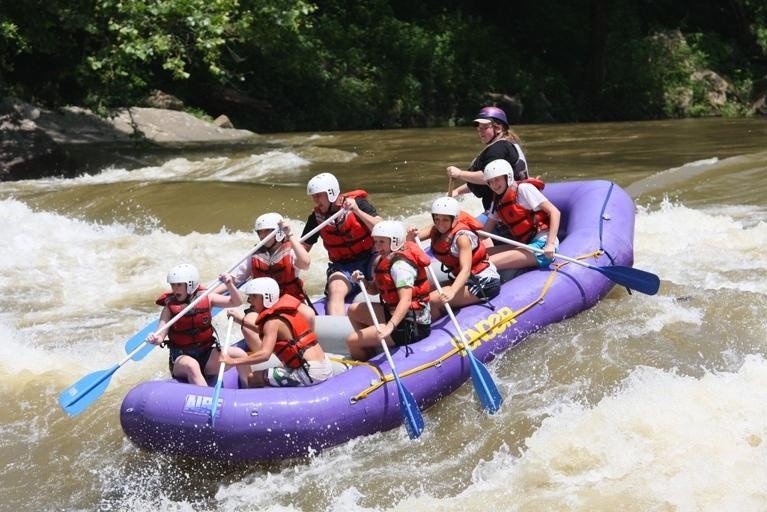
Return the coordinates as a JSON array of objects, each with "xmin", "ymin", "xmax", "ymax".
[
  {"xmin": 284, "ymin": 231, "xmax": 293, "ymax": 239},
  {"xmin": 389, "ymin": 321, "xmax": 396, "ymax": 330}
]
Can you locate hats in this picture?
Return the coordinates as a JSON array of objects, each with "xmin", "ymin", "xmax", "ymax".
[{"xmin": 474, "ymin": 117, "xmax": 494, "ymax": 125}]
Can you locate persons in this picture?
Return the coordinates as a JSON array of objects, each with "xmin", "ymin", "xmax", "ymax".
[
  {"xmin": 348, "ymin": 220, "xmax": 432, "ymax": 361},
  {"xmin": 472, "ymin": 159, "xmax": 559, "ymax": 271},
  {"xmin": 405, "ymin": 196, "xmax": 501, "ymax": 324},
  {"xmin": 445, "ymin": 106, "xmax": 529, "ymax": 216},
  {"xmin": 217, "ymin": 277, "xmax": 333, "ymax": 387},
  {"xmin": 146, "ymin": 263, "xmax": 245, "ymax": 386},
  {"xmin": 213, "ymin": 212, "xmax": 319, "ymax": 316},
  {"xmin": 299, "ymin": 171, "xmax": 384, "ymax": 316}
]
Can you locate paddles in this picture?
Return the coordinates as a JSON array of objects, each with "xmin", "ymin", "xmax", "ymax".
[
  {"xmin": 58, "ymin": 280, "xmax": 223, "ymax": 417},
  {"xmin": 125, "ymin": 228, "xmax": 279, "ymax": 361},
  {"xmin": 477, "ymin": 230, "xmax": 660, "ymax": 295},
  {"xmin": 210, "ymin": 315, "xmax": 234, "ymax": 428},
  {"xmin": 358, "ymin": 272, "xmax": 425, "ymax": 440},
  {"xmin": 210, "ymin": 209, "xmax": 344, "ymax": 316},
  {"xmin": 414, "ymin": 236, "xmax": 504, "ymax": 415}
]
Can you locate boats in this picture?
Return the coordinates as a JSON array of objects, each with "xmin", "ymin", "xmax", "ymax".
[{"xmin": 115, "ymin": 175, "xmax": 638, "ymax": 467}]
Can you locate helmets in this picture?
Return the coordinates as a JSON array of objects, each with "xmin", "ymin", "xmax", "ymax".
[
  {"xmin": 371, "ymin": 220, "xmax": 407, "ymax": 252},
  {"xmin": 246, "ymin": 278, "xmax": 279, "ymax": 308},
  {"xmin": 430, "ymin": 197, "xmax": 459, "ymax": 228},
  {"xmin": 307, "ymin": 172, "xmax": 340, "ymax": 203},
  {"xmin": 255, "ymin": 211, "xmax": 287, "ymax": 242},
  {"xmin": 477, "ymin": 106, "xmax": 510, "ymax": 128},
  {"xmin": 484, "ymin": 159, "xmax": 515, "ymax": 187},
  {"xmin": 167, "ymin": 264, "xmax": 200, "ymax": 295}
]
